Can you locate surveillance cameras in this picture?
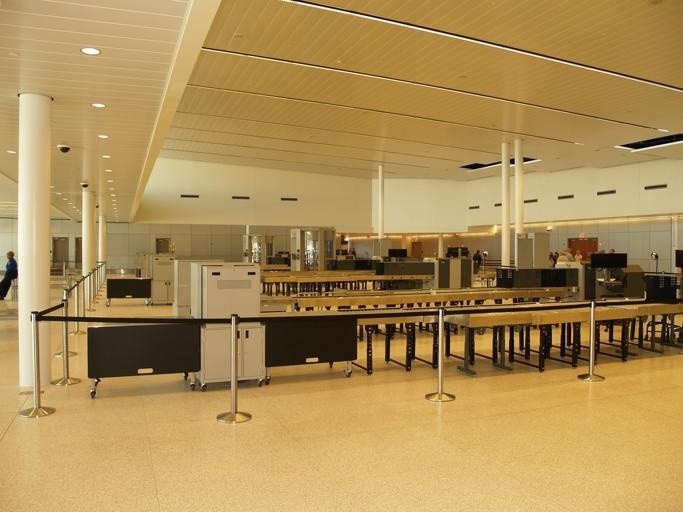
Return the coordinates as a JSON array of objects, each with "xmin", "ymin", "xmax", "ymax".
[
  {"xmin": 82, "ymin": 183, "xmax": 89, "ymax": 188},
  {"xmin": 94, "ymin": 203, "xmax": 99, "ymax": 208},
  {"xmin": 59, "ymin": 145, "xmax": 71, "ymax": 153}
]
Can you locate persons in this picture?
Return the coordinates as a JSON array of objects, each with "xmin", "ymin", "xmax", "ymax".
[
  {"xmin": 559, "ymin": 252, "xmax": 566, "ymax": 260},
  {"xmin": 352, "ymin": 248, "xmax": 356, "ymax": 258},
  {"xmin": 1, "ymin": 251, "xmax": 18, "ymax": 302},
  {"xmin": 550, "ymin": 251, "xmax": 554, "ymax": 268},
  {"xmin": 564, "ymin": 250, "xmax": 573, "ymax": 262},
  {"xmin": 575, "ymin": 250, "xmax": 582, "ymax": 264},
  {"xmin": 473, "ymin": 249, "xmax": 482, "ymax": 272},
  {"xmin": 555, "ymin": 253, "xmax": 558, "ymax": 262}
]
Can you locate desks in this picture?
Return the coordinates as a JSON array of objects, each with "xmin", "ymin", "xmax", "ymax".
[
  {"xmin": 260, "ymin": 270, "xmax": 433, "ymax": 295},
  {"xmin": 339, "ymin": 302, "xmax": 683, "ymax": 378},
  {"xmin": 299, "ymin": 288, "xmax": 579, "ymax": 340}
]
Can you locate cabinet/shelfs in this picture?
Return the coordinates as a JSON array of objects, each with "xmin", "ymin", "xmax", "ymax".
[
  {"xmin": 148, "ymin": 253, "xmax": 173, "ymax": 306},
  {"xmin": 187, "ymin": 259, "xmax": 273, "ymax": 393}
]
